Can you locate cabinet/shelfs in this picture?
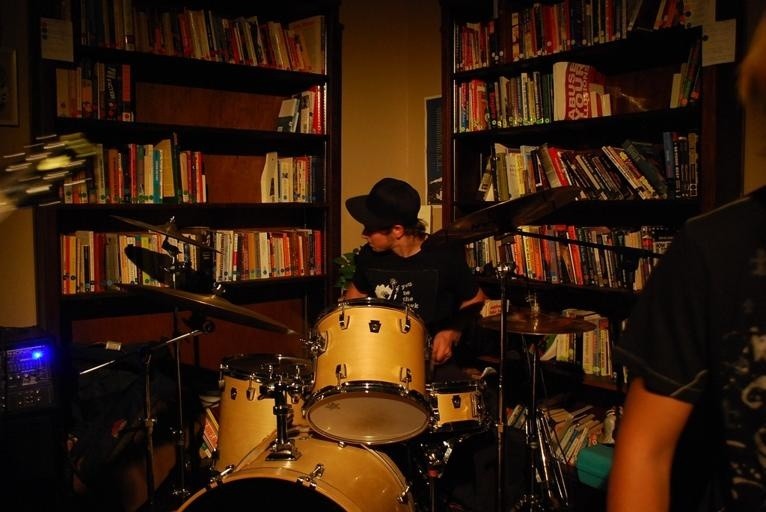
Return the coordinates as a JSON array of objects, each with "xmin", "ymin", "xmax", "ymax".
[
  {"xmin": 445, "ymin": 5, "xmax": 745, "ymax": 512},
  {"xmin": 26, "ymin": 0, "xmax": 342, "ymax": 398}
]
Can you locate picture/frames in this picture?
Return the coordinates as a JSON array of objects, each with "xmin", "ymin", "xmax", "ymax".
[{"xmin": 0, "ymin": 47, "xmax": 20, "ymax": 127}]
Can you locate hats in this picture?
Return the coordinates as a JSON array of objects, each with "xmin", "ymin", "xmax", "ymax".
[{"xmin": 345, "ymin": 178, "xmax": 420, "ymax": 229}]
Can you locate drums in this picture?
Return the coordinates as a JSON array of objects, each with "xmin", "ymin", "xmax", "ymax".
[
  {"xmin": 211, "ymin": 353, "xmax": 313, "ymax": 475},
  {"xmin": 178, "ymin": 428, "xmax": 414, "ymax": 512},
  {"xmin": 424, "ymin": 379, "xmax": 487, "ymax": 433},
  {"xmin": 304, "ymin": 297, "xmax": 430, "ymax": 445}
]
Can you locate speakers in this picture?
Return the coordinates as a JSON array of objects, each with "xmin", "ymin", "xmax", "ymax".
[{"xmin": 443, "ymin": 425, "xmax": 532, "ymax": 511}]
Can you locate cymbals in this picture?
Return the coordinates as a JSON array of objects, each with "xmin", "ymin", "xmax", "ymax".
[
  {"xmin": 116, "ymin": 214, "xmax": 224, "ymax": 254},
  {"xmin": 116, "ymin": 282, "xmax": 289, "ymax": 335},
  {"xmin": 420, "ymin": 185, "xmax": 581, "ymax": 253},
  {"xmin": 125, "ymin": 246, "xmax": 212, "ymax": 295},
  {"xmin": 478, "ymin": 311, "xmax": 597, "ymax": 336}
]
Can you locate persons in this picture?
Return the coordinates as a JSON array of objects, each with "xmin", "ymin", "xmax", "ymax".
[
  {"xmin": 341, "ymin": 175, "xmax": 485, "ymax": 380},
  {"xmin": 603, "ymin": 12, "xmax": 766, "ymax": 512}
]
